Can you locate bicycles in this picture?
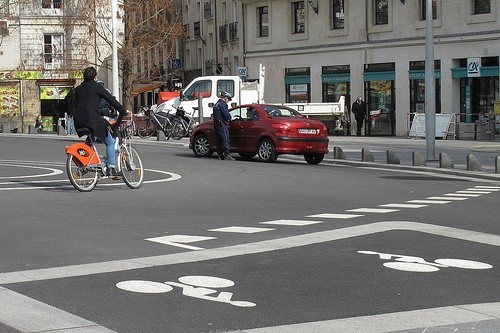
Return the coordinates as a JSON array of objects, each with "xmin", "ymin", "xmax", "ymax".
[
  {"xmin": 137, "ymin": 103, "xmax": 199, "ymax": 140},
  {"xmin": 65, "ymin": 113, "xmax": 144, "ymax": 192}
]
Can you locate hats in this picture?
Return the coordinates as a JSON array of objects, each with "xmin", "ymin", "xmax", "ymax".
[{"xmin": 221, "ymin": 91, "xmax": 232, "ymax": 101}]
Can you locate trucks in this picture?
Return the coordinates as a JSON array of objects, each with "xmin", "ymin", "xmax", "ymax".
[{"xmin": 152, "ymin": 76, "xmax": 345, "ymax": 137}]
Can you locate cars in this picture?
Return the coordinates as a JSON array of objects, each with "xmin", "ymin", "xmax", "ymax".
[{"xmin": 190, "ymin": 104, "xmax": 329, "ymax": 165}]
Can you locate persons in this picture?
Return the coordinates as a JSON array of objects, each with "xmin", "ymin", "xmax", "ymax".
[
  {"xmin": 35, "ymin": 115, "xmax": 44, "ymax": 131},
  {"xmin": 352, "ymin": 96, "xmax": 366, "ymax": 137},
  {"xmin": 233, "ymin": 108, "xmax": 253, "ymax": 120},
  {"xmin": 64, "ymin": 79, "xmax": 118, "ymax": 143},
  {"xmin": 73, "ymin": 66, "xmax": 132, "ymax": 179},
  {"xmin": 212, "ymin": 91, "xmax": 236, "ymax": 161}
]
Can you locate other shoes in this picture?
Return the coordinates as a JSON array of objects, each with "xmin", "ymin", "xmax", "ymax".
[
  {"xmin": 218, "ymin": 155, "xmax": 224, "ymax": 160},
  {"xmin": 105, "ymin": 166, "xmax": 124, "ymax": 179},
  {"xmin": 224, "ymin": 154, "xmax": 236, "ymax": 161}
]
